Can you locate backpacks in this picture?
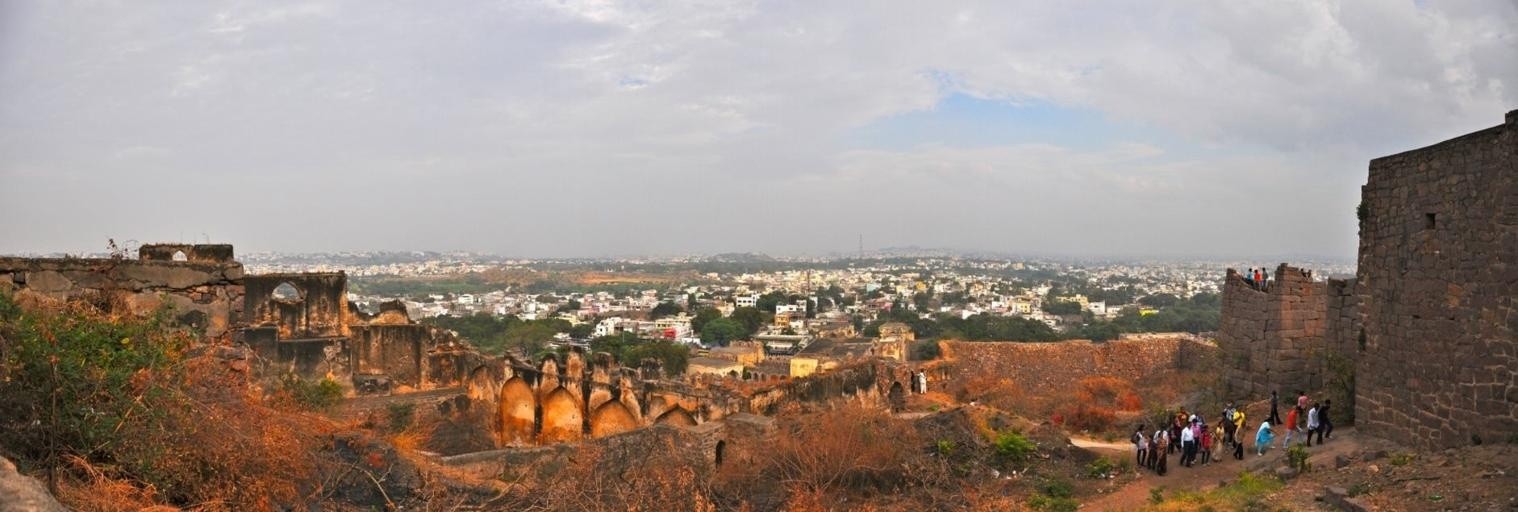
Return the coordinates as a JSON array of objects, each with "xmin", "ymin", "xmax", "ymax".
[{"xmin": 1131, "ymin": 434, "xmax": 1140, "ymax": 443}]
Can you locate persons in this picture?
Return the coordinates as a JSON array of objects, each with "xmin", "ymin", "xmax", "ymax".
[
  {"xmin": 1255, "ymin": 415, "xmax": 1277, "ymax": 457},
  {"xmin": 1300, "ymin": 268, "xmax": 1306, "ymax": 278},
  {"xmin": 1283, "ymin": 405, "xmax": 1305, "ymax": 451},
  {"xmin": 1246, "ymin": 268, "xmax": 1253, "ymax": 286},
  {"xmin": 1131, "ymin": 400, "xmax": 1248, "ymax": 477},
  {"xmin": 1297, "ymin": 391, "xmax": 1309, "ymax": 416},
  {"xmin": 909, "ymin": 371, "xmax": 915, "ymax": 392},
  {"xmin": 1261, "ymin": 267, "xmax": 1269, "ymax": 292},
  {"xmin": 919, "ymin": 367, "xmax": 928, "ymax": 395},
  {"xmin": 1253, "ymin": 270, "xmax": 1262, "ymax": 292},
  {"xmin": 1307, "ymin": 269, "xmax": 1311, "ymax": 279},
  {"xmin": 1307, "ymin": 403, "xmax": 1323, "ymax": 448},
  {"xmin": 1317, "ymin": 399, "xmax": 1334, "ymax": 443},
  {"xmin": 1269, "ymin": 390, "xmax": 1283, "ymax": 426}
]
